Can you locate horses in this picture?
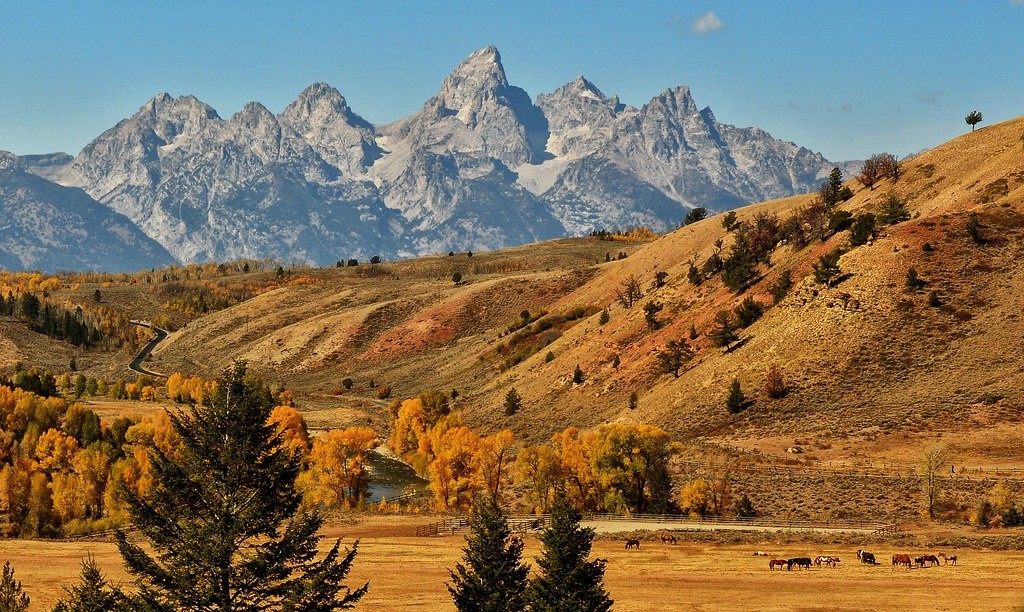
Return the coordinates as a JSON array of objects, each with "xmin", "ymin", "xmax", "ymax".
[
  {"xmin": 661, "ymin": 534, "xmax": 677, "ymax": 545},
  {"xmin": 769, "ymin": 556, "xmax": 836, "ymax": 571},
  {"xmin": 857, "ymin": 549, "xmax": 957, "ymax": 568},
  {"xmin": 625, "ymin": 540, "xmax": 639, "ymax": 550}
]
